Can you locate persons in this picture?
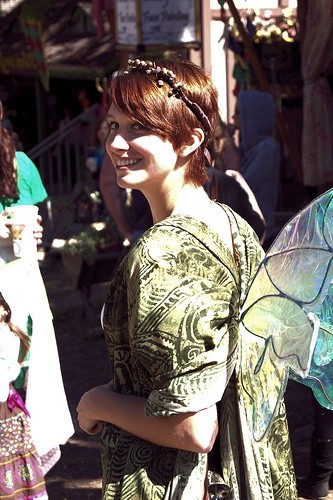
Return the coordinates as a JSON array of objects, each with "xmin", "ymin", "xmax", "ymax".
[
  {"xmin": 76, "ymin": 56, "xmax": 295, "ymax": 500},
  {"xmin": 0, "ymin": 91, "xmax": 333, "ymax": 500}
]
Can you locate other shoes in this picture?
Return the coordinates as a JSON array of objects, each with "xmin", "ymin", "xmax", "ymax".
[{"xmin": 297, "ymin": 476, "xmax": 330, "ymax": 499}]
